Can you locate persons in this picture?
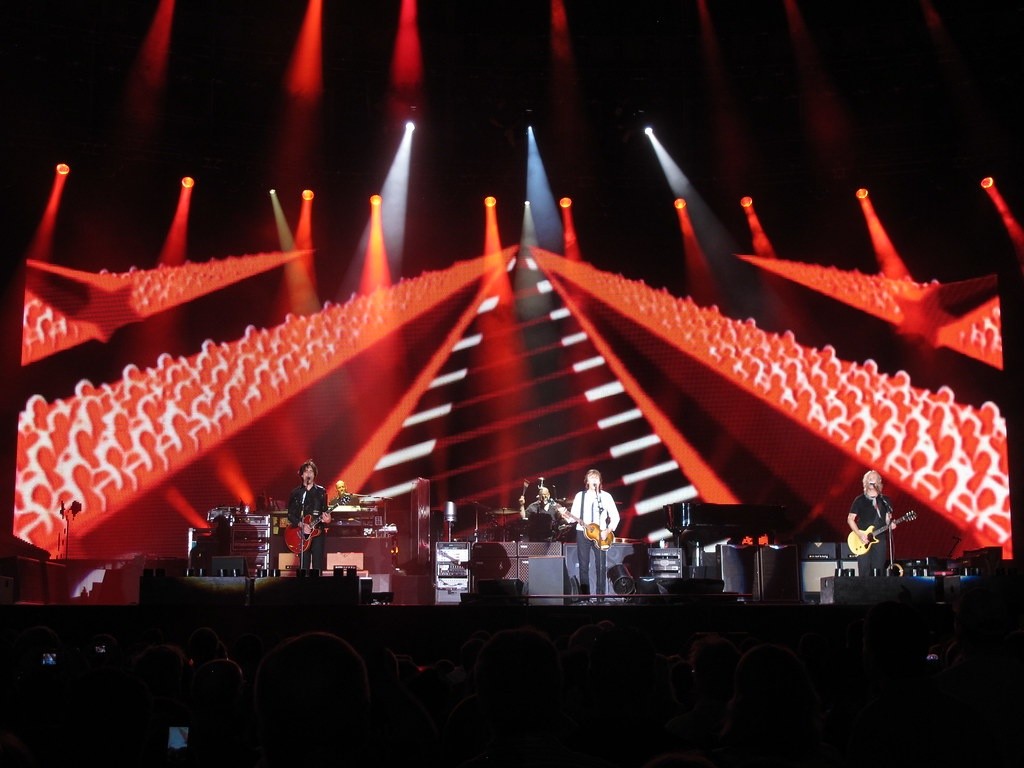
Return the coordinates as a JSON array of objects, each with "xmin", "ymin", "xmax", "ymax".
[
  {"xmin": 519, "ymin": 469, "xmax": 620, "ymax": 604},
  {"xmin": 331, "ymin": 480, "xmax": 349, "ymax": 505},
  {"xmin": 848, "ymin": 470, "xmax": 896, "ymax": 576},
  {"xmin": 287, "ymin": 461, "xmax": 331, "ymax": 575},
  {"xmin": 190, "ymin": 513, "xmax": 230, "ymax": 577},
  {"xmin": 1, "ymin": 585, "xmax": 1024, "ymax": 768}
]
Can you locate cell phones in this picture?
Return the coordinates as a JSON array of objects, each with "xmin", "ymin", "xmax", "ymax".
[
  {"xmin": 42, "ymin": 653, "xmax": 57, "ymax": 665},
  {"xmin": 95, "ymin": 645, "xmax": 106, "ymax": 653},
  {"xmin": 166, "ymin": 724, "xmax": 190, "ymax": 750}
]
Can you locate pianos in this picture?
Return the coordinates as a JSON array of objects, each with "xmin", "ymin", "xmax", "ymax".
[{"xmin": 662, "ymin": 503, "xmax": 790, "ymax": 564}]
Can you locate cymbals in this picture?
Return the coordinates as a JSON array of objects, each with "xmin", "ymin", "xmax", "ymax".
[{"xmin": 487, "ymin": 507, "xmax": 520, "ymax": 514}]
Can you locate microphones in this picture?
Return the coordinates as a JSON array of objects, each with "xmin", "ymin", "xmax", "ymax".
[
  {"xmin": 306, "ymin": 477, "xmax": 310, "ymax": 485},
  {"xmin": 341, "ymin": 491, "xmax": 352, "ymax": 496}
]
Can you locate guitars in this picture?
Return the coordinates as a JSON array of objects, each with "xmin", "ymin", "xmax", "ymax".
[
  {"xmin": 284, "ymin": 494, "xmax": 351, "ymax": 554},
  {"xmin": 550, "ymin": 497, "xmax": 616, "ymax": 552},
  {"xmin": 847, "ymin": 509, "xmax": 917, "ymax": 556}
]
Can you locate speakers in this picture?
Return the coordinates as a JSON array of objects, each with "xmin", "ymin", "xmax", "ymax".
[
  {"xmin": 253, "ymin": 576, "xmax": 359, "ymax": 608},
  {"xmin": 944, "ymin": 577, "xmax": 1024, "ymax": 605},
  {"xmin": 563, "ymin": 543, "xmax": 649, "ymax": 603},
  {"xmin": 760, "ymin": 545, "xmax": 799, "ymax": 602},
  {"xmin": 721, "ymin": 545, "xmax": 761, "ymax": 601},
  {"xmin": 139, "ymin": 576, "xmax": 247, "ymax": 611},
  {"xmin": 821, "ymin": 576, "xmax": 944, "ymax": 605}
]
